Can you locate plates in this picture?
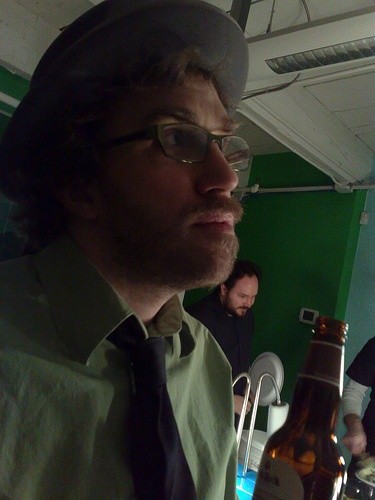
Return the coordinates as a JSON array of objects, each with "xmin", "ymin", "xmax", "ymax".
[{"xmin": 247, "ymin": 351, "xmax": 284, "ymax": 407}]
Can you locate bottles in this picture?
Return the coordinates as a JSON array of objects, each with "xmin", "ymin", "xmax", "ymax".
[{"xmin": 253, "ymin": 316, "xmax": 347, "ymax": 500}]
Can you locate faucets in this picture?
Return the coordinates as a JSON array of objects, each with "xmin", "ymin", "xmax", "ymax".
[
  {"xmin": 243, "ymin": 373, "xmax": 282, "ymax": 475},
  {"xmin": 232, "ymin": 373, "xmax": 250, "ymax": 449}
]
